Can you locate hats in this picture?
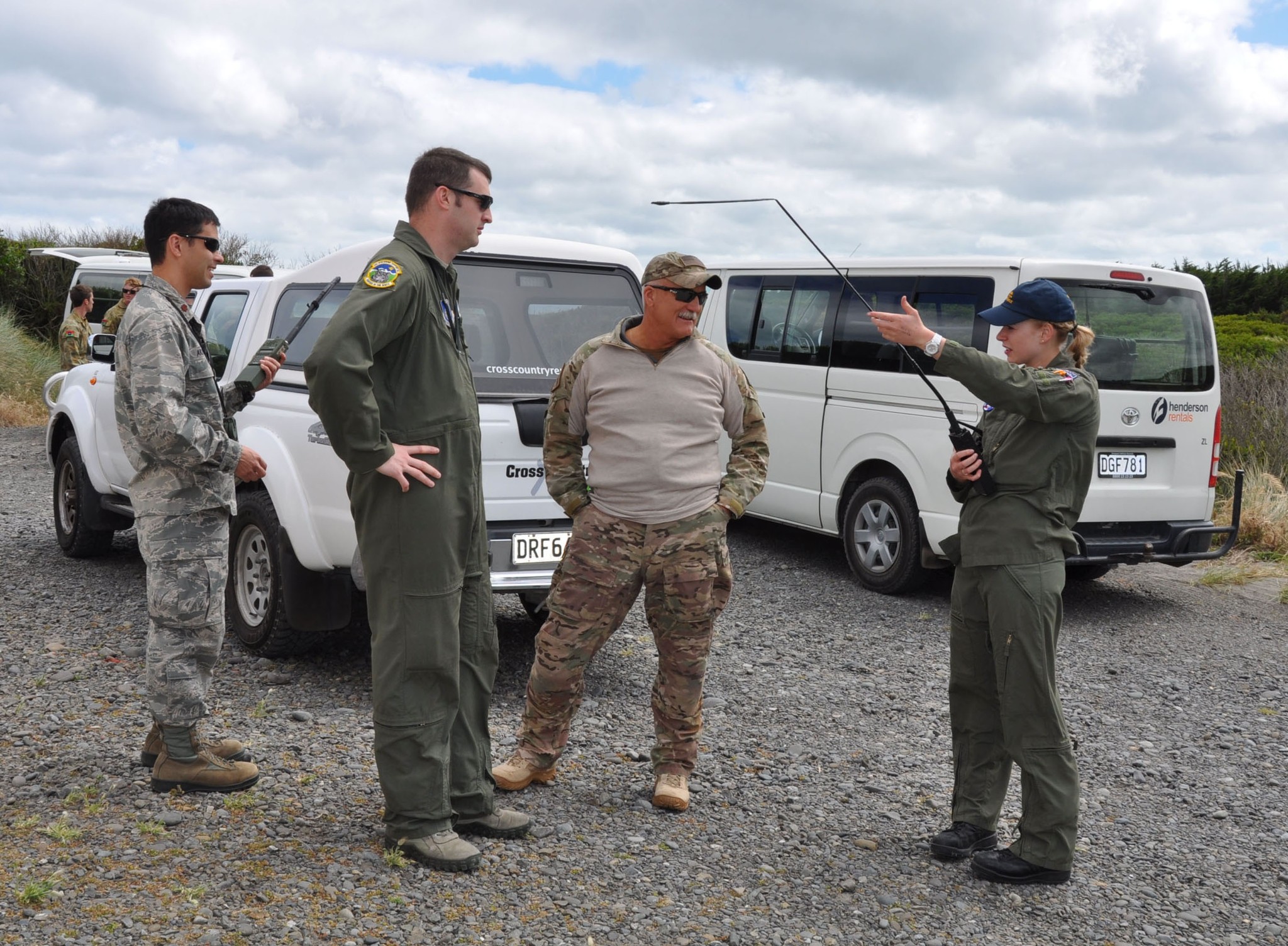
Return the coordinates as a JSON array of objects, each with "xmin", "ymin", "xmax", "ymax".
[
  {"xmin": 977, "ymin": 279, "xmax": 1075, "ymax": 326},
  {"xmin": 642, "ymin": 252, "xmax": 722, "ymax": 289}
]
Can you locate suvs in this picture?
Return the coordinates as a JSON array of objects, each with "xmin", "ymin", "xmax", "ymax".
[{"xmin": 44, "ymin": 233, "xmax": 659, "ymax": 661}]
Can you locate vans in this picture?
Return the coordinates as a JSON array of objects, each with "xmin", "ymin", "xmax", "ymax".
[
  {"xmin": 29, "ymin": 241, "xmax": 294, "ymax": 370},
  {"xmin": 696, "ymin": 257, "xmax": 1245, "ymax": 598}
]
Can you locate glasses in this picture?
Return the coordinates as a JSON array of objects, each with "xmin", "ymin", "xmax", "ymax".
[
  {"xmin": 434, "ymin": 183, "xmax": 493, "ymax": 209},
  {"xmin": 178, "ymin": 234, "xmax": 220, "ymax": 253},
  {"xmin": 645, "ymin": 285, "xmax": 708, "ymax": 305},
  {"xmin": 122, "ymin": 288, "xmax": 139, "ymax": 294}
]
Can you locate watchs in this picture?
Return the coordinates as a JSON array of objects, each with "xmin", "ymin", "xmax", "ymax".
[{"xmin": 923, "ymin": 333, "xmax": 943, "ymax": 358}]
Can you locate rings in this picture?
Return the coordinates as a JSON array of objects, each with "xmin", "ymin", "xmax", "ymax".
[{"xmin": 962, "ymin": 470, "xmax": 969, "ymax": 475}]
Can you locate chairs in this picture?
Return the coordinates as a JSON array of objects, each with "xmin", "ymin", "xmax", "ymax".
[{"xmin": 1069, "ymin": 334, "xmax": 1137, "ymax": 383}]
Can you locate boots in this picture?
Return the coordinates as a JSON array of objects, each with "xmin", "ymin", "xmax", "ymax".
[
  {"xmin": 141, "ymin": 716, "xmax": 244, "ymax": 768},
  {"xmin": 151, "ymin": 721, "xmax": 259, "ymax": 792}
]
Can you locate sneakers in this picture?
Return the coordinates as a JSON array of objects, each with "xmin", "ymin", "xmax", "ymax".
[
  {"xmin": 930, "ymin": 821, "xmax": 998, "ymax": 857},
  {"xmin": 971, "ymin": 848, "xmax": 1071, "ymax": 885},
  {"xmin": 452, "ymin": 806, "xmax": 531, "ymax": 839},
  {"xmin": 491, "ymin": 754, "xmax": 556, "ymax": 791},
  {"xmin": 652, "ymin": 774, "xmax": 689, "ymax": 811},
  {"xmin": 384, "ymin": 827, "xmax": 482, "ymax": 872}
]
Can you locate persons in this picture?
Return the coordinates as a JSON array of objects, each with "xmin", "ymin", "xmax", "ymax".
[
  {"xmin": 101, "ymin": 277, "xmax": 142, "ymax": 337},
  {"xmin": 111, "ymin": 197, "xmax": 287, "ymax": 796},
  {"xmin": 57, "ymin": 284, "xmax": 93, "ymax": 373},
  {"xmin": 301, "ymin": 145, "xmax": 537, "ymax": 872},
  {"xmin": 863, "ymin": 276, "xmax": 1101, "ymax": 886},
  {"xmin": 490, "ymin": 252, "xmax": 772, "ymax": 814}
]
